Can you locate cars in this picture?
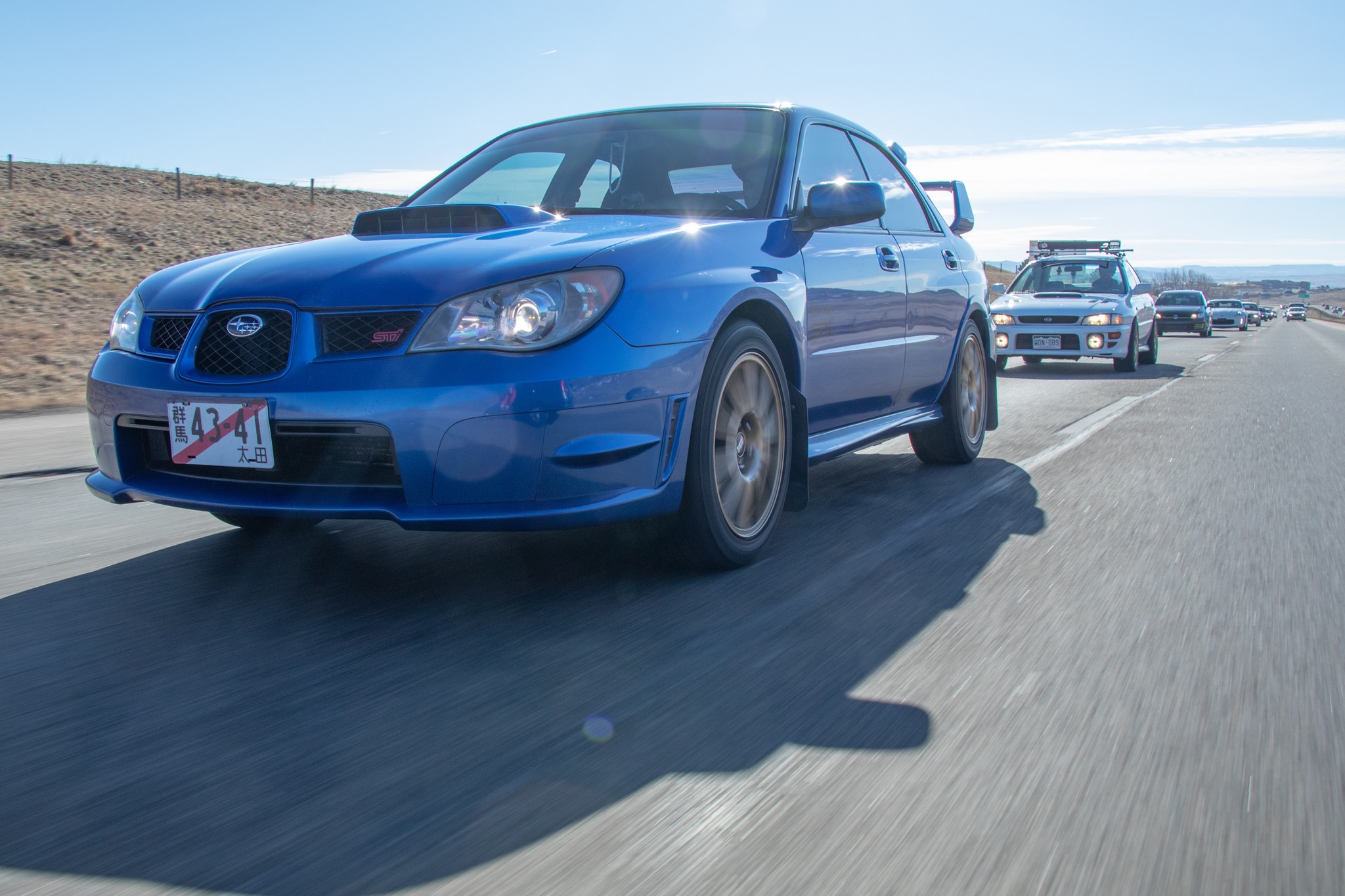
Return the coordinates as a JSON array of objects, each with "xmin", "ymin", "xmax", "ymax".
[
  {"xmin": 1241, "ymin": 302, "xmax": 1261, "ymax": 327},
  {"xmin": 1321, "ymin": 303, "xmax": 1342, "ymax": 314},
  {"xmin": 1280, "ymin": 303, "xmax": 1309, "ymax": 321},
  {"xmin": 1205, "ymin": 299, "xmax": 1248, "ymax": 331},
  {"xmin": 1153, "ymin": 289, "xmax": 1214, "ymax": 338},
  {"xmin": 84, "ymin": 103, "xmax": 997, "ymax": 572},
  {"xmin": 1258, "ymin": 307, "xmax": 1278, "ymax": 322},
  {"xmin": 988, "ymin": 240, "xmax": 1158, "ymax": 373},
  {"xmin": 1342, "ymin": 311, "xmax": 1345, "ymax": 318},
  {"xmin": 1306, "ymin": 302, "xmax": 1310, "ymax": 306}
]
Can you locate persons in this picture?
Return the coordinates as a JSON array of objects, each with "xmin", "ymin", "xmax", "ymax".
[
  {"xmin": 731, "ymin": 146, "xmax": 773, "ymax": 210},
  {"xmin": 1092, "ymin": 263, "xmax": 1119, "ymax": 291}
]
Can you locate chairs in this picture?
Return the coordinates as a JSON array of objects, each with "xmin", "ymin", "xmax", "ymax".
[
  {"xmin": 600, "ymin": 147, "xmax": 676, "ymax": 209},
  {"xmin": 1043, "ymin": 280, "xmax": 1064, "ymax": 292}
]
